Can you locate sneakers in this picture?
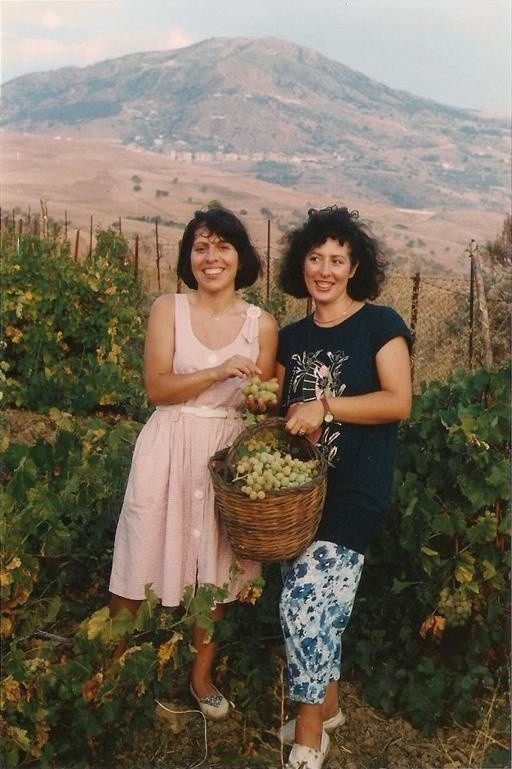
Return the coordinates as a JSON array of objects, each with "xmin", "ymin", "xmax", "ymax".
[
  {"xmin": 191, "ymin": 678, "xmax": 232, "ymax": 721},
  {"xmin": 278, "ymin": 706, "xmax": 347, "ymax": 768}
]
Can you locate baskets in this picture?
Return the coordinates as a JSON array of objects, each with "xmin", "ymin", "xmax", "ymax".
[{"xmin": 207, "ymin": 418, "xmax": 327, "ymax": 562}]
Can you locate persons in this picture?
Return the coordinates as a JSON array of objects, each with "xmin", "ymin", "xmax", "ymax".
[
  {"xmin": 99, "ymin": 207, "xmax": 278, "ymax": 723},
  {"xmin": 272, "ymin": 204, "xmax": 417, "ymax": 769}
]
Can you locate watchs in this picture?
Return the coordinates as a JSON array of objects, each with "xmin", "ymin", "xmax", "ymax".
[{"xmin": 318, "ymin": 394, "xmax": 334, "ymax": 425}]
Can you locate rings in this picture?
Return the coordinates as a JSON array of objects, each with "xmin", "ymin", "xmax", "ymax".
[{"xmin": 298, "ymin": 428, "xmax": 307, "ymax": 435}]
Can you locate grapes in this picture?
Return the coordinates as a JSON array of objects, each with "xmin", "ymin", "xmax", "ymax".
[
  {"xmin": 234, "ymin": 376, "xmax": 319, "ymax": 500},
  {"xmin": 478, "ymin": 509, "xmax": 498, "ymax": 542},
  {"xmin": 438, "ymin": 589, "xmax": 474, "ymax": 628}
]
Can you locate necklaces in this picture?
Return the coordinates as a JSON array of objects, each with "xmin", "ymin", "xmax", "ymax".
[
  {"xmin": 312, "ymin": 298, "xmax": 355, "ymax": 325},
  {"xmin": 198, "ymin": 295, "xmax": 236, "ymax": 322}
]
